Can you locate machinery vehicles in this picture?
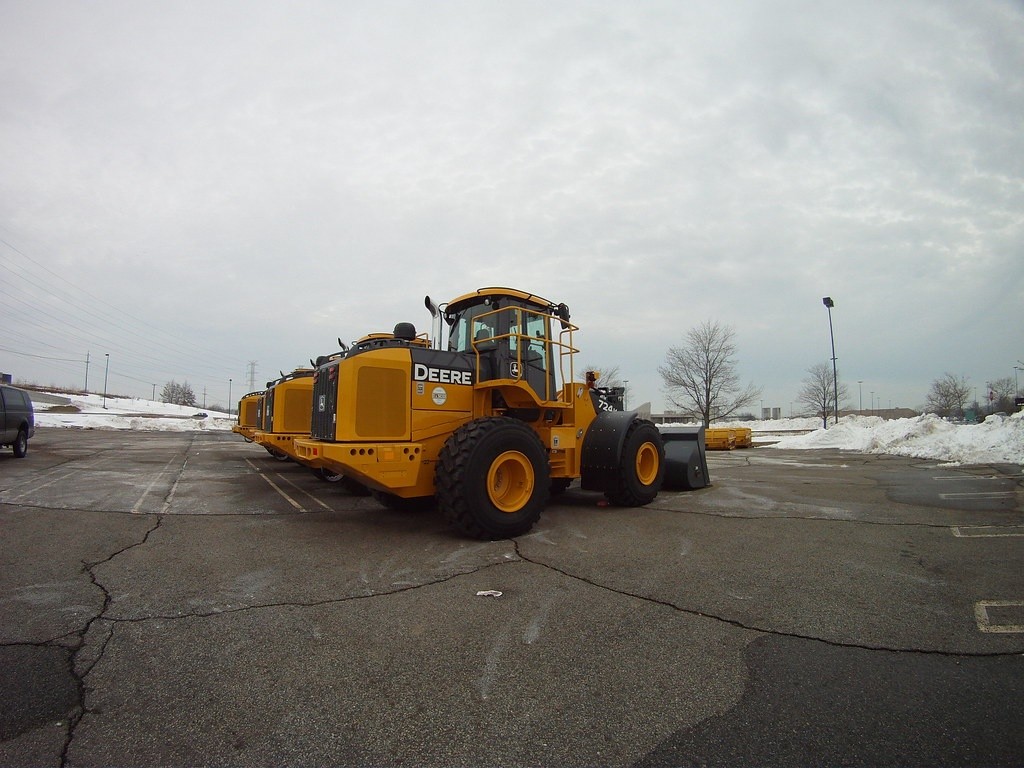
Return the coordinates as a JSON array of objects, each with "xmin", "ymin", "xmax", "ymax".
[{"xmin": 231, "ymin": 287, "xmax": 714, "ymax": 540}]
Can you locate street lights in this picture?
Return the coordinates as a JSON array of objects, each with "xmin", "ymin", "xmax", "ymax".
[
  {"xmin": 858, "ymin": 380, "xmax": 862, "ymax": 416},
  {"xmin": 104, "ymin": 352, "xmax": 109, "ymax": 408},
  {"xmin": 824, "ymin": 297, "xmax": 841, "ymax": 422},
  {"xmin": 229, "ymin": 379, "xmax": 232, "ymax": 418},
  {"xmin": 870, "ymin": 392, "xmax": 874, "ymax": 416},
  {"xmin": 623, "ymin": 381, "xmax": 629, "ymax": 412},
  {"xmin": 1014, "ymin": 367, "xmax": 1018, "ymax": 398}
]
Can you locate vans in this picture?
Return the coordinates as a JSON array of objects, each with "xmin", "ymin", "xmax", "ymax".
[{"xmin": 0, "ymin": 385, "xmax": 35, "ymax": 458}]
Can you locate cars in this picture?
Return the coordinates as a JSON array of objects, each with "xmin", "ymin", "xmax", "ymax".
[{"xmin": 194, "ymin": 413, "xmax": 208, "ymax": 417}]
